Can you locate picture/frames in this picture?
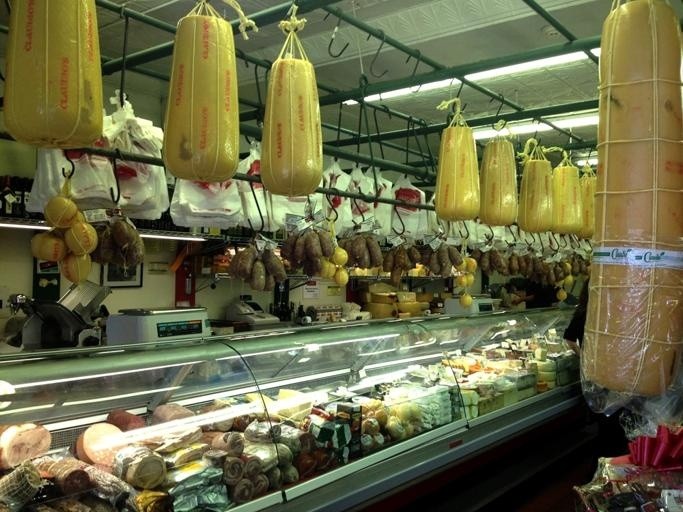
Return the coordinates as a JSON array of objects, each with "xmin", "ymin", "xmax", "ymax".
[{"xmin": 100, "ymin": 261, "xmax": 144, "ymax": 289}]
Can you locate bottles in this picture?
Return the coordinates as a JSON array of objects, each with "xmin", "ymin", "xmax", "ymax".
[{"xmin": 270, "ymin": 302, "xmax": 305, "ymax": 321}]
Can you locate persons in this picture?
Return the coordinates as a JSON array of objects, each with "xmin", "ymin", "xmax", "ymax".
[
  {"xmin": 498, "ymin": 273, "xmax": 554, "ymax": 309},
  {"xmin": 561, "ymin": 270, "xmax": 591, "ymax": 362}
]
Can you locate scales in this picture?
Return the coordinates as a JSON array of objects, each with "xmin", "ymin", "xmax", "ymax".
[
  {"xmin": 105, "ymin": 307, "xmax": 212, "ymax": 346},
  {"xmin": 444, "ymin": 295, "xmax": 503, "ymax": 315}
]
